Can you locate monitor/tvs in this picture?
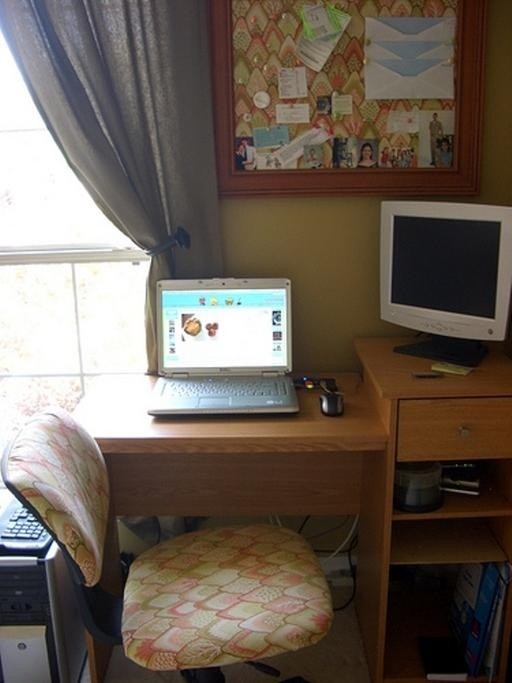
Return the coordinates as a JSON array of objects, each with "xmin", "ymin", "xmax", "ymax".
[{"xmin": 379, "ymin": 201, "xmax": 512, "ymax": 367}]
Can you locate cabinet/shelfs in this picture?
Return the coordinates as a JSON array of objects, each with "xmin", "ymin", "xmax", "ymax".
[{"xmin": 351, "ymin": 334, "xmax": 512, "ymax": 682}]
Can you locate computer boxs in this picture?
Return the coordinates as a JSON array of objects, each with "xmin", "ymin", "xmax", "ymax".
[{"xmin": 0, "ymin": 539, "xmax": 89, "ymax": 683}]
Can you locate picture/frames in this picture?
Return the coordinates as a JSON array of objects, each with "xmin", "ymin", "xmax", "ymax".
[{"xmin": 207, "ymin": 0, "xmax": 486, "ymax": 200}]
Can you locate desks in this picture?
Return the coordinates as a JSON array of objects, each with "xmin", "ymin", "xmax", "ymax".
[{"xmin": 69, "ymin": 372, "xmax": 389, "ymax": 682}]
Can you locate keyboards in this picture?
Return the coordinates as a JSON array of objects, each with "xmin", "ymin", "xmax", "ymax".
[{"xmin": 0, "ymin": 495, "xmax": 53, "ymax": 557}]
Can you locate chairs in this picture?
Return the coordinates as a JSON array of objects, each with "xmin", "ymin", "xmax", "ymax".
[{"xmin": 1, "ymin": 403, "xmax": 332, "ymax": 682}]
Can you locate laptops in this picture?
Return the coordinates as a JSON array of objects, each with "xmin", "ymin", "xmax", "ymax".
[{"xmin": 146, "ymin": 278, "xmax": 300, "ymax": 416}]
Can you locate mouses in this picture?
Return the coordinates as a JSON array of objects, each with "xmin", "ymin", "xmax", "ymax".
[{"xmin": 319, "ymin": 391, "xmax": 345, "ymax": 417}]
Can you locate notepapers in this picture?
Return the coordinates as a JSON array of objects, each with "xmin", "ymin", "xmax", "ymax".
[{"xmin": 431, "ymin": 361, "xmax": 473, "ymax": 375}]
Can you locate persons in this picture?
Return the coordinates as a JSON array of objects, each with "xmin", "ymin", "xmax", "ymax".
[{"xmin": 233, "ymin": 109, "xmax": 454, "ymax": 170}]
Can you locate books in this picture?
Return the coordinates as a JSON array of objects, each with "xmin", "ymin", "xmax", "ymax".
[{"xmin": 416, "ymin": 633, "xmax": 468, "ymax": 681}]
